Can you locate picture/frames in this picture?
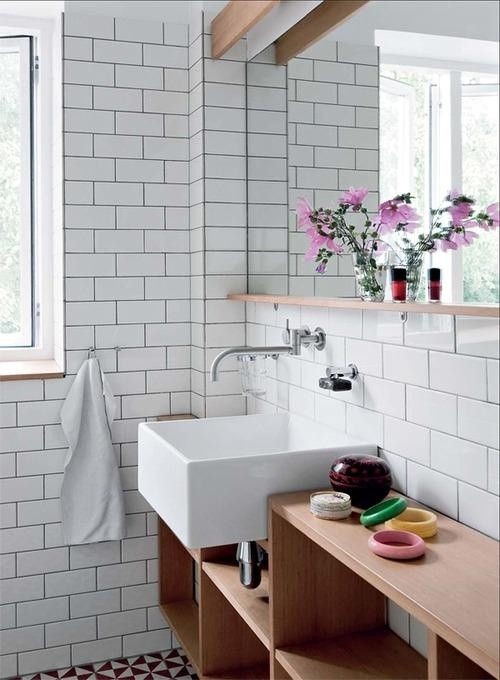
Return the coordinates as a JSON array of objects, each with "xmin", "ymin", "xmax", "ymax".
[{"xmin": 0, "ymin": 6, "xmax": 68, "ymax": 382}]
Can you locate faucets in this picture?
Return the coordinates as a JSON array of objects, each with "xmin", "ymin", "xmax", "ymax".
[{"xmin": 208, "ymin": 326, "xmax": 325, "ymax": 381}]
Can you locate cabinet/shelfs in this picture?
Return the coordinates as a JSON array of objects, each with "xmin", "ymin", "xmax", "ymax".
[{"xmin": 158, "ymin": 411, "xmax": 500, "ymax": 679}]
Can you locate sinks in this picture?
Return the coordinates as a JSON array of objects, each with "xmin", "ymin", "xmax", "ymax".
[{"xmin": 137, "ymin": 410, "xmax": 377, "ymax": 550}]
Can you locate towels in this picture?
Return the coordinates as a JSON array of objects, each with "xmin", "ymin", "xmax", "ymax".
[{"xmin": 58, "ymin": 358, "xmax": 127, "ymax": 547}]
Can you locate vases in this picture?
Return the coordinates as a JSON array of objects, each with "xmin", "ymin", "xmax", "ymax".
[
  {"xmin": 352, "ymin": 250, "xmax": 388, "ymax": 303},
  {"xmin": 397, "ymin": 264, "xmax": 428, "ymax": 305}
]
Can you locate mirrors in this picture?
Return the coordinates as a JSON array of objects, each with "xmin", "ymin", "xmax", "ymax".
[{"xmin": 245, "ymin": 0, "xmax": 500, "ymax": 318}]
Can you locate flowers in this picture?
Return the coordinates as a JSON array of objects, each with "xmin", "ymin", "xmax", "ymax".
[
  {"xmin": 294, "ymin": 184, "xmax": 423, "ymax": 301},
  {"xmin": 393, "ymin": 191, "xmax": 500, "ymax": 297}
]
